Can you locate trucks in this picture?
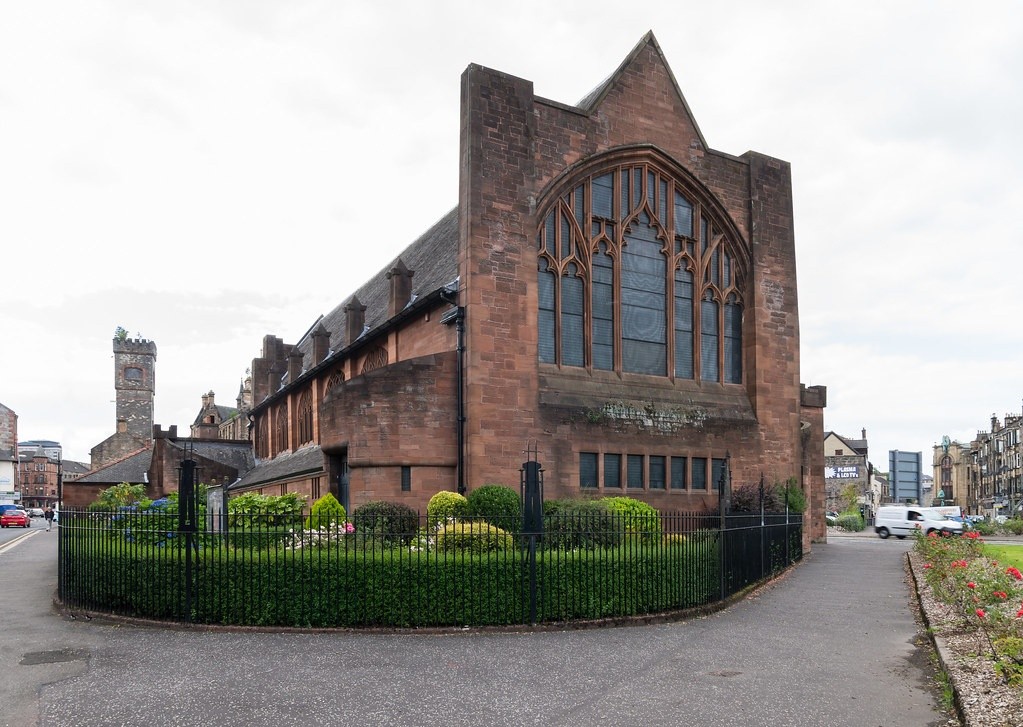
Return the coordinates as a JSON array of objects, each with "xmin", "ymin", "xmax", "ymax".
[{"xmin": 929, "ymin": 506, "xmax": 962, "ymax": 518}]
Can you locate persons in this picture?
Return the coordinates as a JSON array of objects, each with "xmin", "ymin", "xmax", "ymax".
[
  {"xmin": 912, "ymin": 513, "xmax": 918, "ymax": 520},
  {"xmin": 45, "ymin": 507, "xmax": 54, "ymax": 531}
]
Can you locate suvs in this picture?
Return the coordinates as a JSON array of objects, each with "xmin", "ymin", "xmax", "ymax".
[{"xmin": 0, "ymin": 504, "xmax": 26, "ymax": 515}]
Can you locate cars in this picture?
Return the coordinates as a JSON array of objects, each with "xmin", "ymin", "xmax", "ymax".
[
  {"xmin": 944, "ymin": 515, "xmax": 974, "ymax": 531},
  {"xmin": 25, "ymin": 508, "xmax": 45, "ymax": 518},
  {"xmin": 0, "ymin": 509, "xmax": 31, "ymax": 528}
]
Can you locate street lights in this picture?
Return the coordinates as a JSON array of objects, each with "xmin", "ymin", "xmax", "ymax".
[{"xmin": 19, "ymin": 455, "xmax": 27, "ymax": 504}]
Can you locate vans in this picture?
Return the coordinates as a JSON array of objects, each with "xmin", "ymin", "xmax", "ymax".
[{"xmin": 873, "ymin": 505, "xmax": 964, "ymax": 541}]
